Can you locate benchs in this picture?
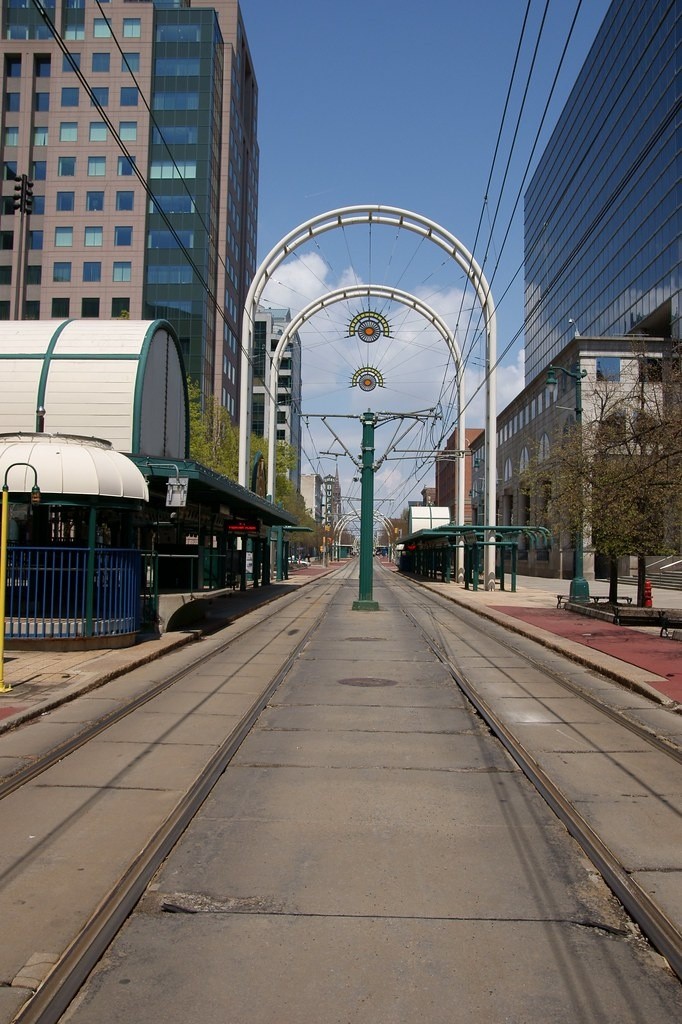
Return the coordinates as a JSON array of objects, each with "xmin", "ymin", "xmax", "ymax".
[
  {"xmin": 611, "ymin": 605, "xmax": 682, "ymax": 626},
  {"xmin": 557, "ymin": 595, "xmax": 632, "ymax": 610}
]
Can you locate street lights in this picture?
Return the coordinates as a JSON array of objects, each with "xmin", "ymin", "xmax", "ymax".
[
  {"xmin": 0, "ymin": 462, "xmax": 41, "ymax": 696},
  {"xmin": 13, "ymin": 173, "xmax": 34, "ymax": 321},
  {"xmin": 544, "ymin": 363, "xmax": 589, "ymax": 602}
]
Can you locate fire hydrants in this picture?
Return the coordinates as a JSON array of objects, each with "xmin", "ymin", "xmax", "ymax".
[{"xmin": 643, "ymin": 579, "xmax": 653, "ymax": 606}]
